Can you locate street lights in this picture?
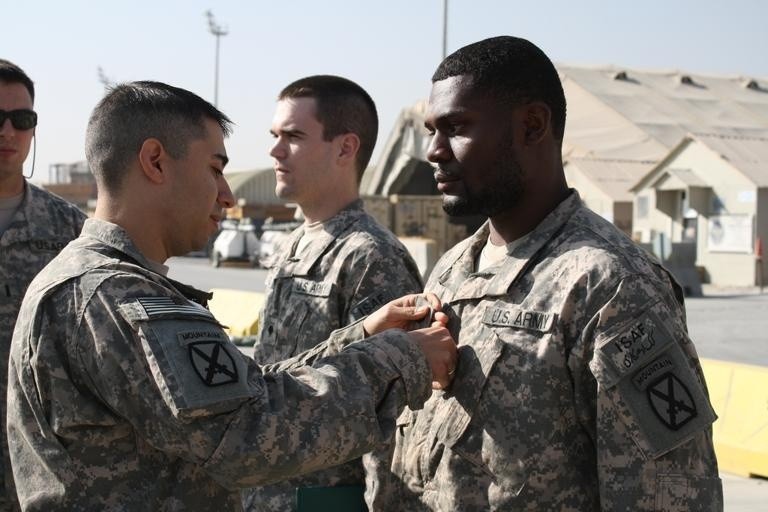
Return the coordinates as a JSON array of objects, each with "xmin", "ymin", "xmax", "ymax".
[{"xmin": 207, "ymin": 10, "xmax": 231, "ymax": 108}]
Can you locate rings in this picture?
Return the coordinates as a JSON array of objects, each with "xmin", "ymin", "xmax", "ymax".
[{"xmin": 447, "ymin": 365, "xmax": 457, "ymax": 379}]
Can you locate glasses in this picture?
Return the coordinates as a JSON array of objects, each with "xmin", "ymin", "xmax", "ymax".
[{"xmin": 0, "ymin": 110, "xmax": 38, "ymax": 131}]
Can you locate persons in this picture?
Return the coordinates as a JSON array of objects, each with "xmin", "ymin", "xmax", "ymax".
[
  {"xmin": 1, "ymin": 55, "xmax": 88, "ymax": 510},
  {"xmin": 247, "ymin": 74, "xmax": 422, "ymax": 512},
  {"xmin": 356, "ymin": 33, "xmax": 725, "ymax": 512},
  {"xmin": 4, "ymin": 79, "xmax": 459, "ymax": 512}
]
